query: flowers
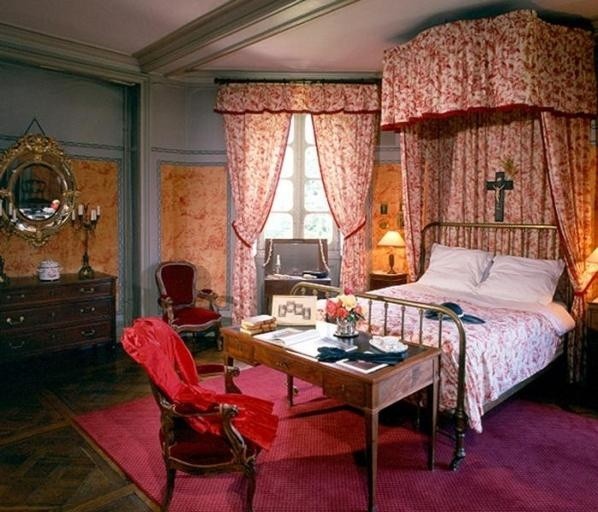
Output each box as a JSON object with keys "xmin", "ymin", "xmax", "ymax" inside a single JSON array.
[{"xmin": 324, "ymin": 287, "xmax": 365, "ymax": 321}]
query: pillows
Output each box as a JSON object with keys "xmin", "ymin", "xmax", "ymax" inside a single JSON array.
[
  {"xmin": 478, "ymin": 251, "xmax": 567, "ymax": 309},
  {"xmin": 414, "ymin": 241, "xmax": 492, "ymax": 292}
]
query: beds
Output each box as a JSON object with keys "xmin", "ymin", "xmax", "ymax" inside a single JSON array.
[{"xmin": 290, "ymin": 220, "xmax": 576, "ymax": 472}]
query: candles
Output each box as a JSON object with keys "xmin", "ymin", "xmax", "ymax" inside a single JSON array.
[
  {"xmin": 90, "ymin": 209, "xmax": 96, "ymax": 219},
  {"xmin": 96, "ymin": 205, "xmax": 100, "ymax": 215},
  {"xmin": 70, "ymin": 208, "xmax": 76, "ymax": 221},
  {"xmin": 78, "ymin": 205, "xmax": 83, "ymax": 215}
]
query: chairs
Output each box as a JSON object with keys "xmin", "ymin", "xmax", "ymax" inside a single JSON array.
[
  {"xmin": 122, "ymin": 316, "xmax": 276, "ymax": 511},
  {"xmin": 152, "ymin": 260, "xmax": 227, "ymax": 351}
]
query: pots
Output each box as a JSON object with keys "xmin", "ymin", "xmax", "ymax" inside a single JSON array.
[{"xmin": 35, "ymin": 259, "xmax": 63, "ymax": 282}]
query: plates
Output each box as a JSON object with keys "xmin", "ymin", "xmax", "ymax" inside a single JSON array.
[{"xmin": 368, "ymin": 335, "xmax": 408, "ymax": 353}]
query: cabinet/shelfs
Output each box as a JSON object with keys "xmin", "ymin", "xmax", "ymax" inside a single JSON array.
[
  {"xmin": 2, "ymin": 271, "xmax": 118, "ymax": 360},
  {"xmin": 263, "ymin": 240, "xmax": 331, "ymax": 314}
]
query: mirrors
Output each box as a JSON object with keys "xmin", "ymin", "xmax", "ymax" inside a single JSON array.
[{"xmin": 1, "ymin": 133, "xmax": 78, "ymax": 245}]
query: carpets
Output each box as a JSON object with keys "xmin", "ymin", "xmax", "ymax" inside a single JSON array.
[{"xmin": 76, "ymin": 359, "xmax": 598, "ymax": 510}]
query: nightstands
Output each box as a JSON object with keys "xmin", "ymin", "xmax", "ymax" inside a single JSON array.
[
  {"xmin": 369, "ymin": 272, "xmax": 406, "ymax": 291},
  {"xmin": 580, "ymin": 302, "xmax": 598, "ymax": 413}
]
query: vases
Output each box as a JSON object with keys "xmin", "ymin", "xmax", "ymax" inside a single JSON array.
[{"xmin": 333, "ymin": 316, "xmax": 356, "ymax": 335}]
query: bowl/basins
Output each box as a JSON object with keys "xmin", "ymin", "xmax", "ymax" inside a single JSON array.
[{"xmin": 383, "ymin": 335, "xmax": 399, "ymax": 348}]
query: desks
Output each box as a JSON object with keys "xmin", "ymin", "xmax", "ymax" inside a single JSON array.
[{"xmin": 224, "ymin": 316, "xmax": 443, "ymax": 510}]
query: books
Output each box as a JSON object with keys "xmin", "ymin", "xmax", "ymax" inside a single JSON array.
[
  {"xmin": 241, "ymin": 323, "xmax": 276, "ymax": 330},
  {"xmin": 241, "ymin": 314, "xmax": 276, "ymax": 326},
  {"xmin": 240, "ymin": 327, "xmax": 277, "ymax": 336}
]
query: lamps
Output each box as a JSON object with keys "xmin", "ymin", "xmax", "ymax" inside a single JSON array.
[
  {"xmin": 377, "ymin": 229, "xmax": 408, "ymax": 275},
  {"xmin": 588, "ymin": 238, "xmax": 598, "ymax": 296}
]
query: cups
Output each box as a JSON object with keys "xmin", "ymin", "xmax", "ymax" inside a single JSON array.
[{"xmin": 372, "ymin": 336, "xmax": 383, "ymax": 346}]
